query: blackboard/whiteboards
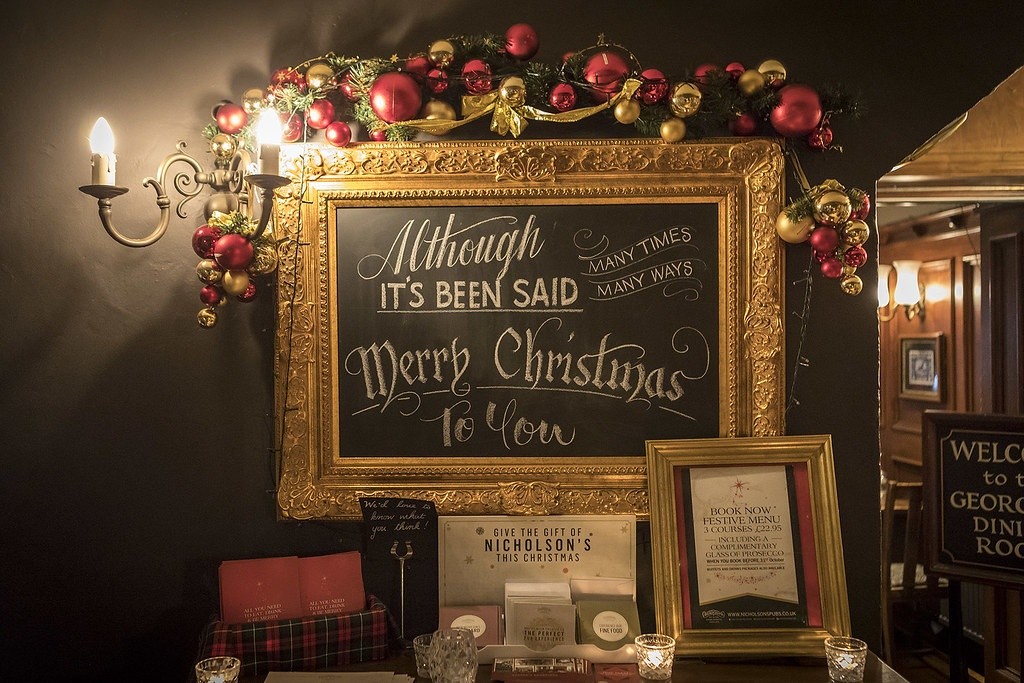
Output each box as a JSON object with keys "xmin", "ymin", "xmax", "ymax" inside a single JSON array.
[{"xmin": 276, "ymin": 139, "xmax": 790, "ymax": 523}]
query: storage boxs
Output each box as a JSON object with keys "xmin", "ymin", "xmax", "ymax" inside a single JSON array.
[{"xmin": 191, "ymin": 599, "xmax": 404, "ymax": 683}]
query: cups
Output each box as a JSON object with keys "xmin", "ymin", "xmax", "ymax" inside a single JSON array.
[
  {"xmin": 196, "ymin": 656, "xmax": 240, "ymax": 683},
  {"xmin": 635, "ymin": 633, "xmax": 675, "ymax": 680},
  {"xmin": 824, "ymin": 636, "xmax": 868, "ymax": 683},
  {"xmin": 413, "ymin": 634, "xmax": 436, "ymax": 679},
  {"xmin": 429, "ymin": 628, "xmax": 478, "ymax": 683}
]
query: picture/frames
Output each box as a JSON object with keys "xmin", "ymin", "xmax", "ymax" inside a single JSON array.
[
  {"xmin": 646, "ymin": 440, "xmax": 853, "ymax": 661},
  {"xmin": 922, "ymin": 409, "xmax": 1024, "ymax": 591},
  {"xmin": 259, "ymin": 132, "xmax": 790, "ymax": 519},
  {"xmin": 896, "ymin": 328, "xmax": 946, "ymax": 404}
]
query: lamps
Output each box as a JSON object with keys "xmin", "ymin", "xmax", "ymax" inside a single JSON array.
[
  {"xmin": 78, "ymin": 98, "xmax": 294, "ymax": 246},
  {"xmin": 874, "ymin": 256, "xmax": 928, "ymax": 321}
]
